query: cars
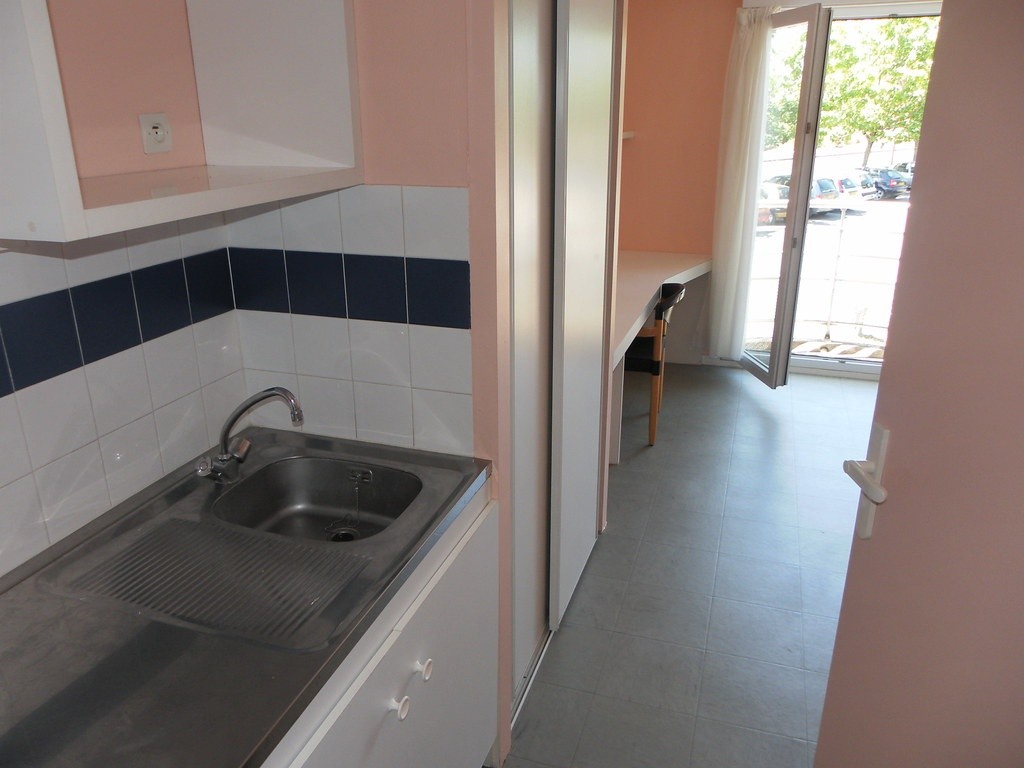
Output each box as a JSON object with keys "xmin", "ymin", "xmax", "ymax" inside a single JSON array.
[{"xmin": 754, "ymin": 159, "xmax": 916, "ymax": 227}]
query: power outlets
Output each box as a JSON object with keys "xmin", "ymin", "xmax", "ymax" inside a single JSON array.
[{"xmin": 139, "ymin": 108, "xmax": 173, "ymax": 156}]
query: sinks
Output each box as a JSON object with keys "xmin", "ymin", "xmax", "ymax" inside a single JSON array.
[{"xmin": 210, "ymin": 456, "xmax": 423, "ymax": 544}]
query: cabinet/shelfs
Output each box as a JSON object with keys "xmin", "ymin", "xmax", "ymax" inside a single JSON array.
[
  {"xmin": 257, "ymin": 482, "xmax": 501, "ymax": 767},
  {"xmin": 0, "ymin": 0, "xmax": 363, "ymax": 242}
]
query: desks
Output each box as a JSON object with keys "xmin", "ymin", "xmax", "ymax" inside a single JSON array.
[{"xmin": 610, "ymin": 249, "xmax": 714, "ymax": 464}]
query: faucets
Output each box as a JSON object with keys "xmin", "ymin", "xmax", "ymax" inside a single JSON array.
[{"xmin": 194, "ymin": 386, "xmax": 305, "ymax": 486}]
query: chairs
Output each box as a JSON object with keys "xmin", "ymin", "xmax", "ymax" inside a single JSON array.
[{"xmin": 624, "ymin": 282, "xmax": 686, "ymax": 446}]
